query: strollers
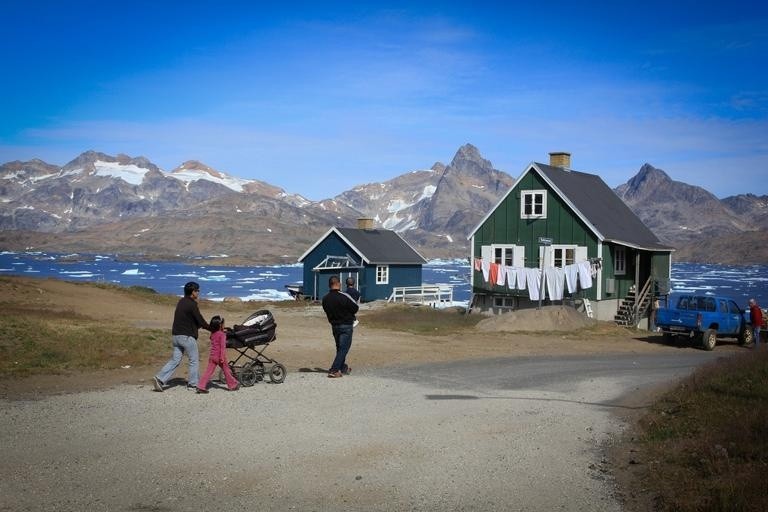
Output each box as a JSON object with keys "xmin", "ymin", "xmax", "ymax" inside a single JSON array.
[{"xmin": 219, "ymin": 310, "xmax": 286, "ymax": 387}]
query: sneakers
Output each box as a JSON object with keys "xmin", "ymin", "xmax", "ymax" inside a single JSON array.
[
  {"xmin": 328, "ymin": 371, "xmax": 342, "ymax": 377},
  {"xmin": 187, "ymin": 383, "xmax": 197, "ymax": 390},
  {"xmin": 346, "ymin": 368, "xmax": 351, "ymax": 373},
  {"xmin": 353, "ymin": 320, "xmax": 359, "ymax": 328},
  {"xmin": 153, "ymin": 376, "xmax": 162, "ymax": 391},
  {"xmin": 197, "ymin": 388, "xmax": 209, "ymax": 393},
  {"xmin": 234, "ymin": 385, "xmax": 239, "ymax": 391}
]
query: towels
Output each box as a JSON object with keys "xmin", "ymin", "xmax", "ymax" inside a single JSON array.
[{"xmin": 474, "ymin": 258, "xmax": 594, "ymax": 301}]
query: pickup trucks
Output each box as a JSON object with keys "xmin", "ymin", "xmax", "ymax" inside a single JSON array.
[{"xmin": 654, "ymin": 294, "xmax": 754, "ymax": 350}]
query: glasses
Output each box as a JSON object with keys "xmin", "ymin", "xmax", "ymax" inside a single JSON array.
[{"xmin": 195, "ymin": 289, "xmax": 200, "ymax": 292}]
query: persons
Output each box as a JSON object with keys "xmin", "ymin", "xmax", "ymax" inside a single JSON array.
[
  {"xmin": 322, "ymin": 275, "xmax": 359, "ymax": 378},
  {"xmin": 344, "ymin": 277, "xmax": 359, "ymax": 327},
  {"xmin": 196, "ymin": 315, "xmax": 241, "ymax": 392},
  {"xmin": 152, "ymin": 281, "xmax": 210, "ymax": 392},
  {"xmin": 749, "ymin": 298, "xmax": 763, "ymax": 346}
]
